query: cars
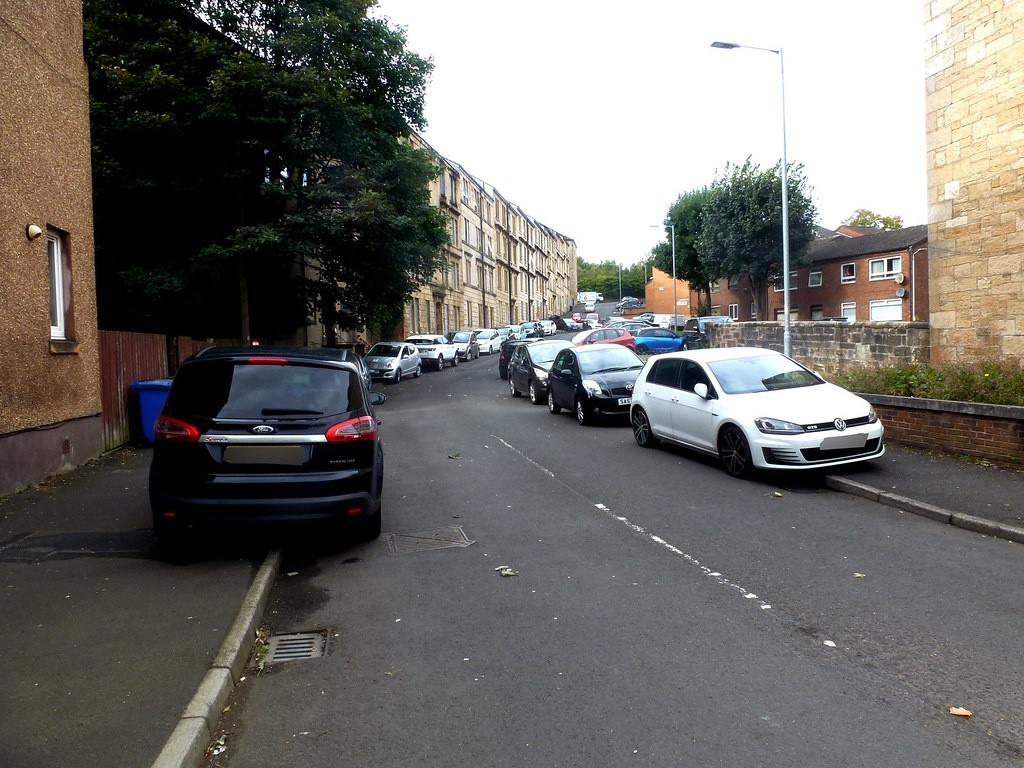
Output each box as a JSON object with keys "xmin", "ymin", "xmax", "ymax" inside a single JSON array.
[
  {"xmin": 519, "ymin": 321, "xmax": 544, "ymax": 338},
  {"xmin": 570, "ymin": 327, "xmax": 635, "ymax": 352},
  {"xmin": 630, "ymin": 346, "xmax": 887, "ymax": 477},
  {"xmin": 363, "ymin": 341, "xmax": 423, "ymax": 384},
  {"xmin": 581, "ymin": 318, "xmax": 604, "ymax": 330},
  {"xmin": 634, "ymin": 327, "xmax": 689, "ymax": 354},
  {"xmin": 507, "ymin": 338, "xmax": 576, "ymax": 404},
  {"xmin": 632, "ymin": 312, "xmax": 654, "ymax": 322},
  {"xmin": 556, "ymin": 319, "xmax": 581, "ymax": 332},
  {"xmin": 614, "ymin": 296, "xmax": 641, "ymax": 311},
  {"xmin": 499, "ymin": 337, "xmax": 547, "ymax": 380},
  {"xmin": 540, "ymin": 320, "xmax": 557, "ymax": 336},
  {"xmin": 601, "ymin": 315, "xmax": 660, "ymax": 331},
  {"xmin": 505, "ymin": 325, "xmax": 527, "ymax": 339},
  {"xmin": 572, "ymin": 312, "xmax": 581, "ymax": 319},
  {"xmin": 472, "ymin": 329, "xmax": 509, "ymax": 354},
  {"xmin": 445, "ymin": 332, "xmax": 481, "ymax": 362},
  {"xmin": 546, "ymin": 343, "xmax": 646, "ymax": 426}
]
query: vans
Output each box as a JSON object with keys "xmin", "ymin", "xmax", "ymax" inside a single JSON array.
[{"xmin": 683, "ymin": 316, "xmax": 734, "ymax": 338}]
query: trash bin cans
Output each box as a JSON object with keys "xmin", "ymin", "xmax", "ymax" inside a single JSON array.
[{"xmin": 131, "ymin": 378, "xmax": 173, "ymax": 446}]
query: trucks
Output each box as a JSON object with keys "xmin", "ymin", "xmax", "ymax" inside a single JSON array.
[
  {"xmin": 577, "ymin": 291, "xmax": 604, "ymax": 306},
  {"xmin": 649, "ymin": 313, "xmax": 684, "ymax": 331}
]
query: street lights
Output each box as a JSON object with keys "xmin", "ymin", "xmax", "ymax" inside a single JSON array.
[
  {"xmin": 911, "ymin": 248, "xmax": 928, "ymax": 322},
  {"xmin": 711, "ymin": 36, "xmax": 794, "ymax": 360},
  {"xmin": 649, "ymin": 224, "xmax": 677, "ymax": 336},
  {"xmin": 642, "ymin": 263, "xmax": 647, "ymax": 284}
]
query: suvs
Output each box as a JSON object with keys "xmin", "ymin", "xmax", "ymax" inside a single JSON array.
[
  {"xmin": 404, "ymin": 334, "xmax": 459, "ymax": 372},
  {"xmin": 147, "ymin": 344, "xmax": 385, "ymax": 545}
]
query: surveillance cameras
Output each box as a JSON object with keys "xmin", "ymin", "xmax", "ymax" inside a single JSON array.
[{"xmin": 28, "ymin": 225, "xmax": 42, "ymax": 239}]
